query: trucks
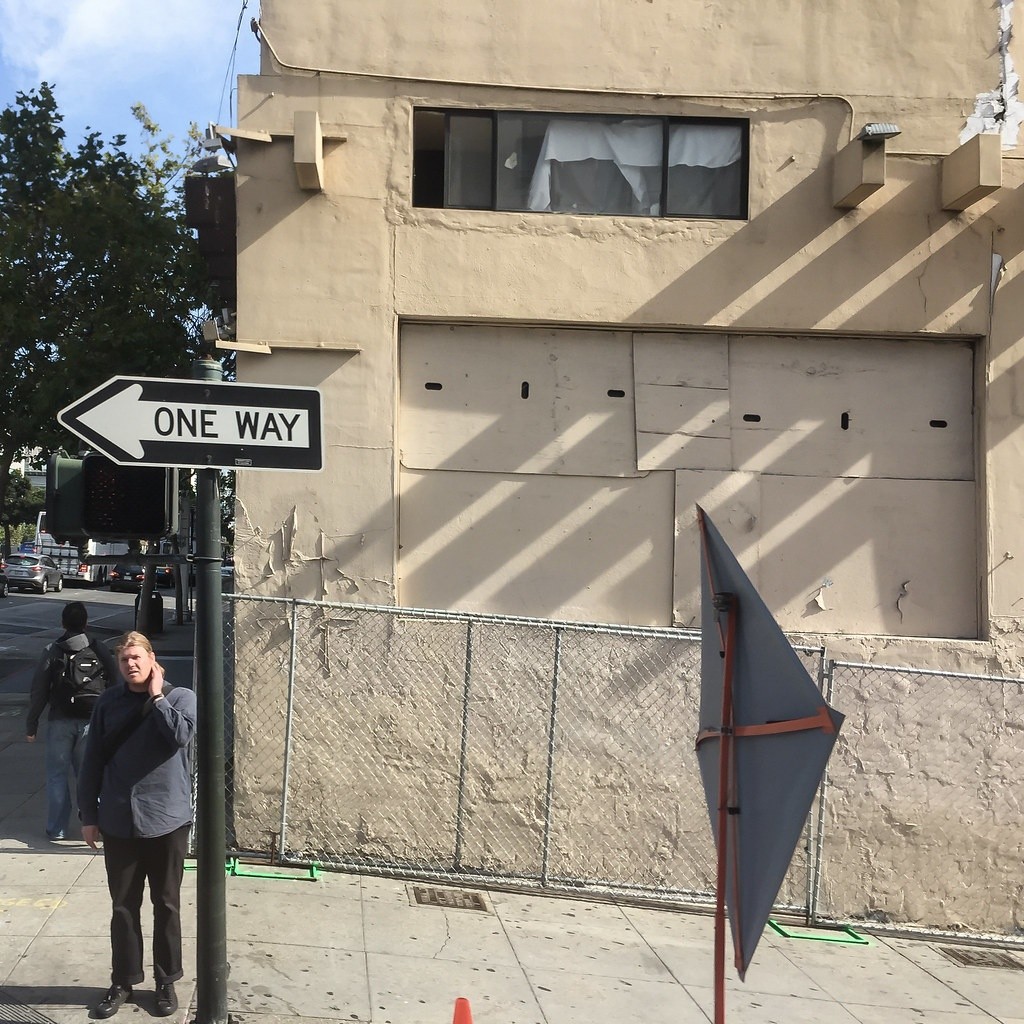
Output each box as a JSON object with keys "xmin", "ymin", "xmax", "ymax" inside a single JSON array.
[{"xmin": 34, "ymin": 511, "xmax": 147, "ymax": 588}]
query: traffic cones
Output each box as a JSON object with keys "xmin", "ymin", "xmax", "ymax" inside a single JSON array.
[{"xmin": 452, "ymin": 998, "xmax": 474, "ymax": 1024}]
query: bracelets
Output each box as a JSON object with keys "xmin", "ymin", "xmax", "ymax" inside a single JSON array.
[{"xmin": 150, "ymin": 693, "xmax": 164, "ymax": 703}]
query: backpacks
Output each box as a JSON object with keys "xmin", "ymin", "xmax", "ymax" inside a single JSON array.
[{"xmin": 53, "ymin": 638, "xmax": 107, "ymax": 712}]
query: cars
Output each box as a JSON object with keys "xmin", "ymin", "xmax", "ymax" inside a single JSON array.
[
  {"xmin": 155, "ymin": 563, "xmax": 177, "ymax": 590},
  {"xmin": 2, "ymin": 553, "xmax": 64, "ymax": 595},
  {"xmin": 110, "ymin": 562, "xmax": 159, "ymax": 593},
  {"xmin": 17, "ymin": 542, "xmax": 34, "ymax": 553},
  {"xmin": 222, "ymin": 557, "xmax": 235, "ymax": 578},
  {"xmin": 0, "ymin": 568, "xmax": 10, "ymax": 598}
]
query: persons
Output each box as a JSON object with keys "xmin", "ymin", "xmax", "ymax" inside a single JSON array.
[
  {"xmin": 79, "ymin": 632, "xmax": 198, "ymax": 1017},
  {"xmin": 26, "ymin": 602, "xmax": 115, "ymax": 840}
]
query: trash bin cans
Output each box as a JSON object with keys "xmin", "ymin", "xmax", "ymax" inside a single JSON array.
[{"xmin": 131, "ymin": 590, "xmax": 163, "ymax": 639}]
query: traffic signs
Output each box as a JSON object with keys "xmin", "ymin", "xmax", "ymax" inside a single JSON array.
[{"xmin": 58, "ymin": 375, "xmax": 326, "ymax": 476}]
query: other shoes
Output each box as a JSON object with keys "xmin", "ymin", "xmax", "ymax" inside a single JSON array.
[
  {"xmin": 155, "ymin": 981, "xmax": 178, "ymax": 1015},
  {"xmin": 96, "ymin": 984, "xmax": 133, "ymax": 1017},
  {"xmin": 46, "ymin": 828, "xmax": 64, "ymax": 840}
]
